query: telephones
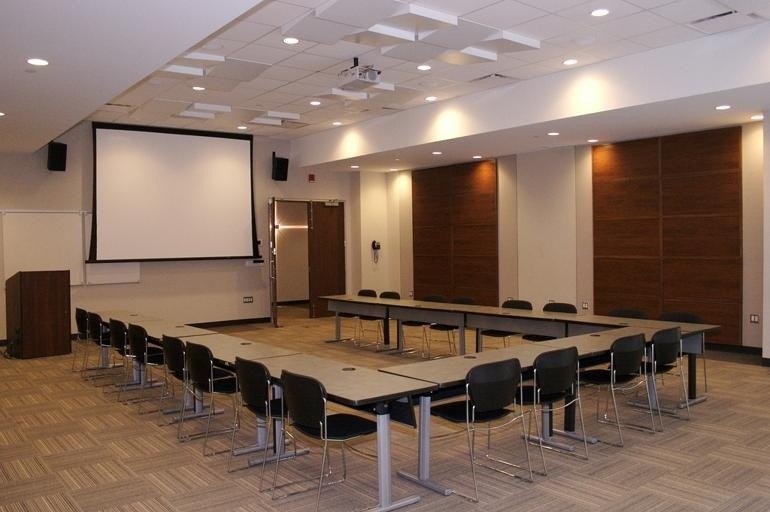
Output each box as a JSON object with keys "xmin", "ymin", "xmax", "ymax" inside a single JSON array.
[{"xmin": 372, "ymin": 240, "xmax": 380, "ymax": 249}]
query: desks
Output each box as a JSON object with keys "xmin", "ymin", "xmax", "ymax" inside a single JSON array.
[
  {"xmin": 378, "ymin": 341, "xmax": 573, "ymax": 497},
  {"xmin": 248, "ymin": 352, "xmax": 440, "ymax": 510}
]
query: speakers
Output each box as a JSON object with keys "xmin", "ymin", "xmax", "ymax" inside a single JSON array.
[
  {"xmin": 272, "ymin": 158, "xmax": 288, "ymax": 181},
  {"xmin": 47, "ymin": 142, "xmax": 67, "ymax": 171}
]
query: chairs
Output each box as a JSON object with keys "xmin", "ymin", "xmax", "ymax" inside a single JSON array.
[
  {"xmin": 230, "ymin": 356, "xmax": 331, "ymax": 493},
  {"xmin": 533, "ymin": 316, "xmax": 720, "ymax": 448},
  {"xmin": 606, "ymin": 307, "xmax": 646, "ymax": 319},
  {"xmin": 488, "ymin": 347, "xmax": 591, "ymax": 478},
  {"xmin": 319, "ymin": 290, "xmax": 577, "ymax": 359},
  {"xmin": 656, "ymin": 310, "xmax": 712, "ymax": 402},
  {"xmin": 430, "ymin": 357, "xmax": 534, "ymax": 503},
  {"xmin": 270, "ymin": 368, "xmax": 379, "ymax": 508},
  {"xmin": 72, "ymin": 309, "xmax": 302, "ymax": 473}
]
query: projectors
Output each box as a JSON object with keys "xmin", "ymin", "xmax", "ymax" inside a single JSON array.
[{"xmin": 337, "ymin": 66, "xmax": 380, "ymax": 91}]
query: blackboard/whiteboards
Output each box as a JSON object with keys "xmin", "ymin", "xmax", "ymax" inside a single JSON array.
[{"xmin": 2, "ymin": 209, "xmax": 144, "ymax": 289}]
